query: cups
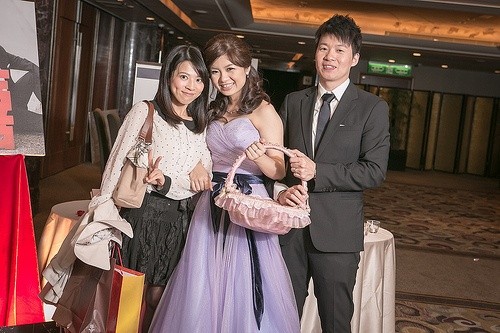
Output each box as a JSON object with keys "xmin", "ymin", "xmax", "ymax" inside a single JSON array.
[
  {"xmin": 367, "ymin": 220, "xmax": 380, "ymax": 233},
  {"xmin": 364, "ymin": 223, "xmax": 369, "ymax": 236}
]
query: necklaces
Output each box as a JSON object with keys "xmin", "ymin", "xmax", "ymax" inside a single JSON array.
[{"xmin": 225, "ymin": 109, "xmax": 239, "ymax": 114}]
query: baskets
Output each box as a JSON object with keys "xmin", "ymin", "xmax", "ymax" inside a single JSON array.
[{"xmin": 214, "ymin": 143, "xmax": 312, "ymax": 236}]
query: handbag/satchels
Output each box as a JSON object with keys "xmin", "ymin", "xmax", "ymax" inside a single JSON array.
[
  {"xmin": 112, "ymin": 99, "xmax": 155, "ymax": 209},
  {"xmin": 106, "ymin": 242, "xmax": 145, "ymax": 333},
  {"xmin": 52, "ymin": 243, "xmax": 116, "ymax": 333}
]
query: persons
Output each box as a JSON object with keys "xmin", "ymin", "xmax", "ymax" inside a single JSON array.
[
  {"xmin": 1, "ymin": 46, "xmax": 43, "ymax": 134},
  {"xmin": 148, "ymin": 34, "xmax": 301, "ymax": 333},
  {"xmin": 88, "ymin": 45, "xmax": 213, "ymax": 333},
  {"xmin": 262, "ymin": 13, "xmax": 390, "ymax": 333}
]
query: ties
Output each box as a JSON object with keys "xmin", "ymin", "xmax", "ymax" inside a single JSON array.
[{"xmin": 314, "ymin": 93, "xmax": 335, "ymax": 159}]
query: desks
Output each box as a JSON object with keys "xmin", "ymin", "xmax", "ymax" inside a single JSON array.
[
  {"xmin": 300, "ymin": 224, "xmax": 395, "ymax": 333},
  {"xmin": 37, "ymin": 200, "xmax": 92, "ymax": 291}
]
